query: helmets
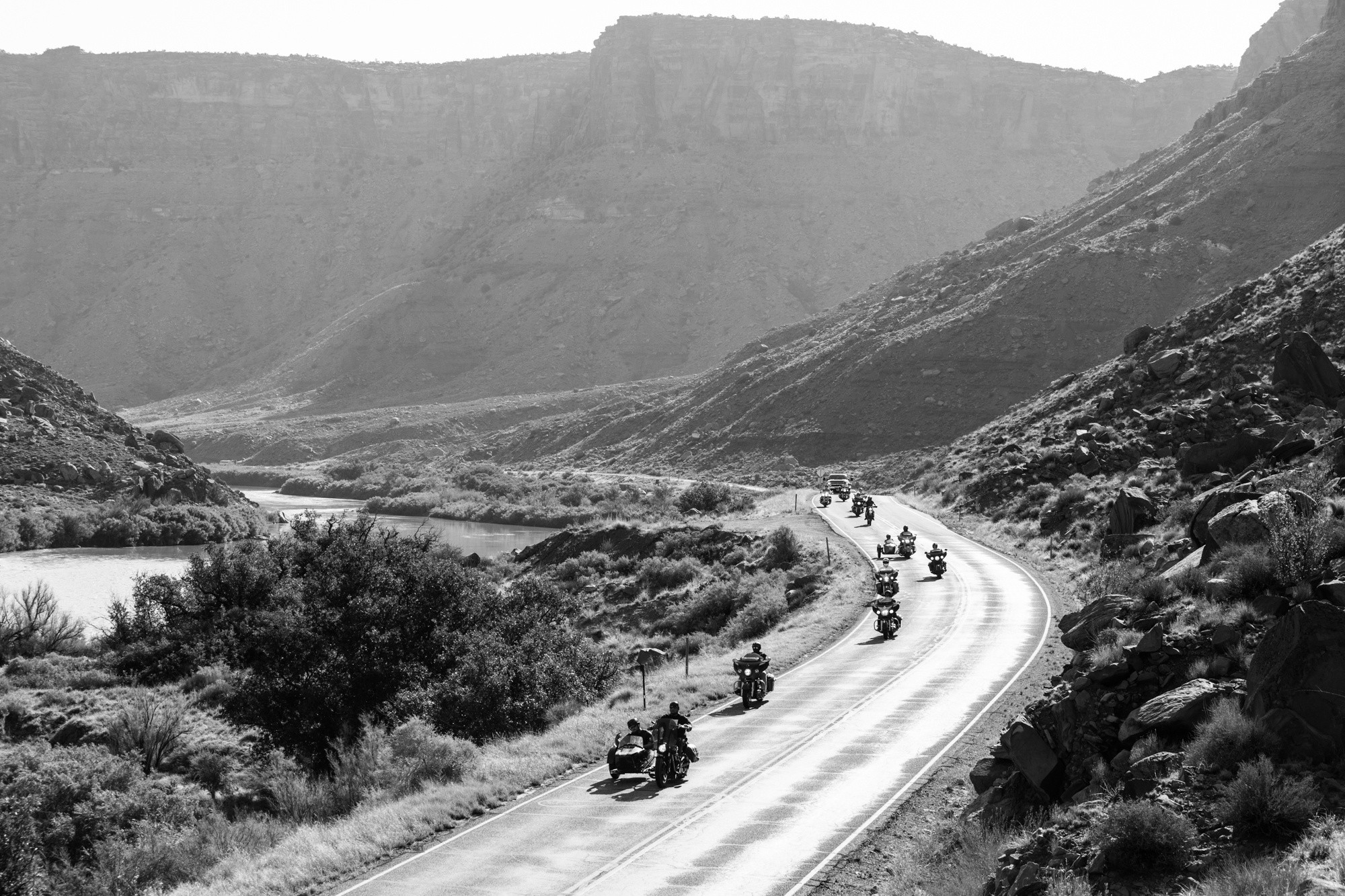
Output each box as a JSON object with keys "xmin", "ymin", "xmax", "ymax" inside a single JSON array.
[
  {"xmin": 628, "ymin": 718, "xmax": 638, "ymax": 728},
  {"xmin": 933, "ymin": 543, "xmax": 938, "ymax": 548},
  {"xmin": 885, "ymin": 590, "xmax": 892, "ymax": 595},
  {"xmin": 670, "ymin": 701, "xmax": 679, "ymax": 706},
  {"xmin": 903, "ymin": 525, "xmax": 908, "ymax": 530},
  {"xmin": 752, "ymin": 642, "xmax": 761, "ymax": 647},
  {"xmin": 883, "ymin": 558, "xmax": 889, "ymax": 561},
  {"xmin": 868, "ymin": 495, "xmax": 872, "ymax": 500},
  {"xmin": 857, "ymin": 492, "xmax": 860, "ymax": 496},
  {"xmin": 886, "ymin": 534, "xmax": 890, "ymax": 537}
]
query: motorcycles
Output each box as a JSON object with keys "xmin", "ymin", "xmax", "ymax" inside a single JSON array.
[
  {"xmin": 877, "ymin": 534, "xmax": 917, "ymax": 559},
  {"xmin": 924, "ymin": 548, "xmax": 947, "ymax": 579},
  {"xmin": 607, "ymin": 721, "xmax": 699, "ymax": 787},
  {"xmin": 864, "ymin": 505, "xmax": 877, "ymax": 526},
  {"xmin": 872, "ymin": 596, "xmax": 901, "ymax": 640},
  {"xmin": 851, "ymin": 492, "xmax": 868, "ymax": 517},
  {"xmin": 820, "ymin": 490, "xmax": 832, "ymax": 508},
  {"xmin": 732, "ymin": 652, "xmax": 777, "ymax": 709},
  {"xmin": 839, "ymin": 486, "xmax": 850, "ymax": 502},
  {"xmin": 875, "ymin": 569, "xmax": 900, "ymax": 598}
]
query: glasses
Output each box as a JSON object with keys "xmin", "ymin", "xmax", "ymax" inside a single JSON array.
[
  {"xmin": 669, "ymin": 706, "xmax": 677, "ymax": 708},
  {"xmin": 753, "ymin": 646, "xmax": 759, "ymax": 649},
  {"xmin": 629, "ymin": 725, "xmax": 637, "ymax": 729}
]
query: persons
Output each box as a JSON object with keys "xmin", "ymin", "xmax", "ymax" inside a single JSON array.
[
  {"xmin": 856, "ymin": 488, "xmax": 865, "ymax": 497},
  {"xmin": 646, "ymin": 700, "xmax": 692, "ymax": 765},
  {"xmin": 872, "ymin": 590, "xmax": 901, "ymax": 632},
  {"xmin": 734, "ymin": 642, "xmax": 770, "ymax": 697},
  {"xmin": 852, "ymin": 493, "xmax": 863, "ymax": 505},
  {"xmin": 614, "ymin": 717, "xmax": 654, "ymax": 759},
  {"xmin": 820, "ymin": 484, "xmax": 831, "ymax": 504},
  {"xmin": 875, "ymin": 557, "xmax": 900, "ymax": 592},
  {"xmin": 928, "ymin": 543, "xmax": 947, "ymax": 572},
  {"xmin": 898, "ymin": 525, "xmax": 916, "ymax": 554},
  {"xmin": 864, "ymin": 495, "xmax": 876, "ymax": 521},
  {"xmin": 885, "ymin": 534, "xmax": 894, "ymax": 544},
  {"xmin": 839, "ymin": 481, "xmax": 852, "ymax": 493}
]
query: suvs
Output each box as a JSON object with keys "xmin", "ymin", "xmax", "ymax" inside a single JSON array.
[{"xmin": 823, "ymin": 473, "xmax": 853, "ymax": 494}]
